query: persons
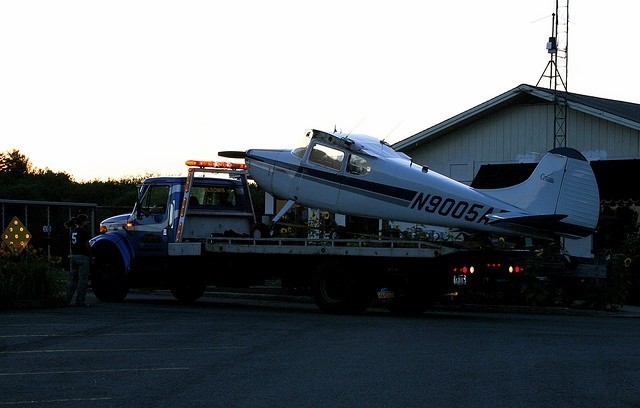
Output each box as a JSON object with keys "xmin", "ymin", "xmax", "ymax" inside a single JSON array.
[
  {"xmin": 64, "ymin": 213, "xmax": 91, "ymax": 308},
  {"xmin": 218, "ymin": 190, "xmax": 233, "ymax": 206}
]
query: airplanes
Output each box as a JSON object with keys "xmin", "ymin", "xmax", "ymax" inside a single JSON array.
[{"xmin": 245, "ymin": 127, "xmax": 601, "ymax": 248}]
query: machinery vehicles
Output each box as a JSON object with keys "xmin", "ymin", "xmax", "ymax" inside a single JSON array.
[{"xmin": 88, "ymin": 160, "xmax": 528, "ymax": 314}]
